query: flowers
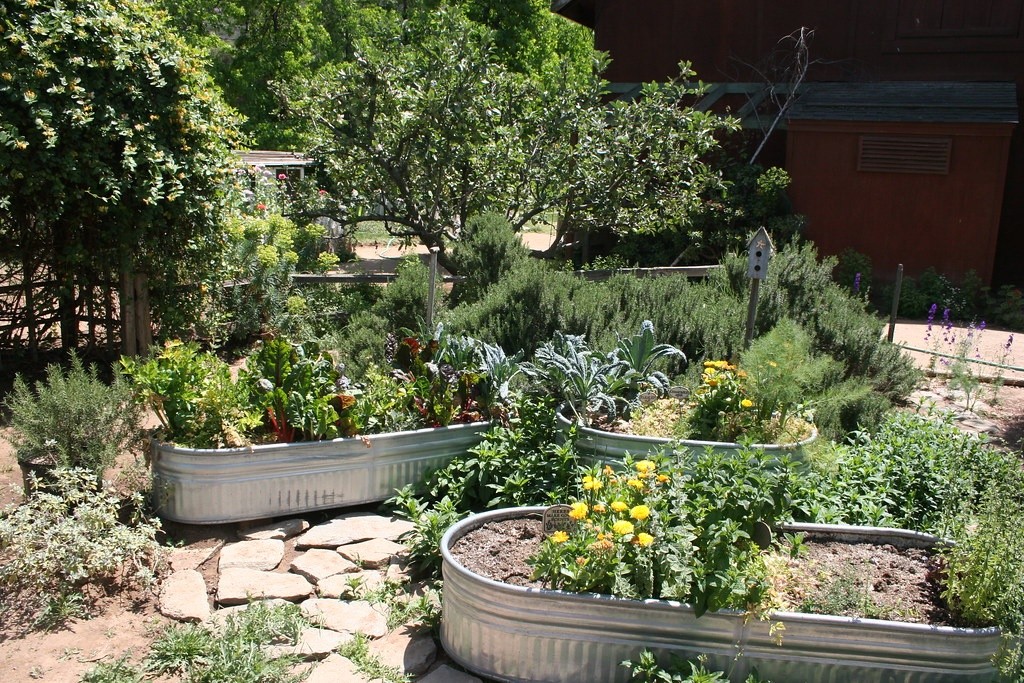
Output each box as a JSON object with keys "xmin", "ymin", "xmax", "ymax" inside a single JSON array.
[{"xmin": 133, "ymin": 312, "xmax": 803, "ymax": 635}]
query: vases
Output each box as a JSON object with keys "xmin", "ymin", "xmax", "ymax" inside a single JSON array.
[
  {"xmin": 439, "ymin": 504, "xmax": 1024, "ymax": 683},
  {"xmin": 556, "ymin": 390, "xmax": 819, "ymax": 476},
  {"xmin": 143, "ymin": 416, "xmax": 494, "ymax": 528}
]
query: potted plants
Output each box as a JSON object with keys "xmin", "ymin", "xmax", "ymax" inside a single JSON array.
[{"xmin": 7, "ymin": 353, "xmax": 122, "ymax": 518}]
